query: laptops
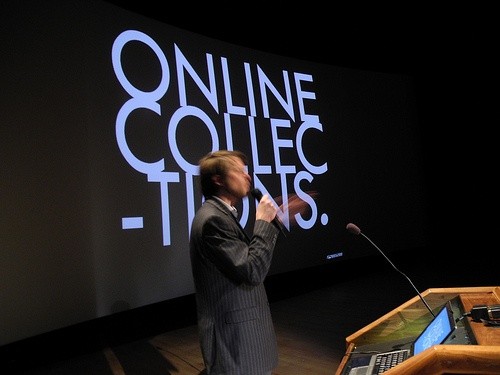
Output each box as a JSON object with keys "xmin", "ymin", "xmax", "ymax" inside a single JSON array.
[{"xmin": 366, "ymin": 302, "xmax": 457, "ymax": 375}]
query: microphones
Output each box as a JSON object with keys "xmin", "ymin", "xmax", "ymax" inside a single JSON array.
[
  {"xmin": 345, "ymin": 222, "xmax": 436, "ymax": 318},
  {"xmin": 251, "ymin": 189, "xmax": 288, "ymax": 239}
]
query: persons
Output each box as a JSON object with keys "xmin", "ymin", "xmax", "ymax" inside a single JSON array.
[{"xmin": 188, "ymin": 149, "xmax": 320, "ymax": 375}]
